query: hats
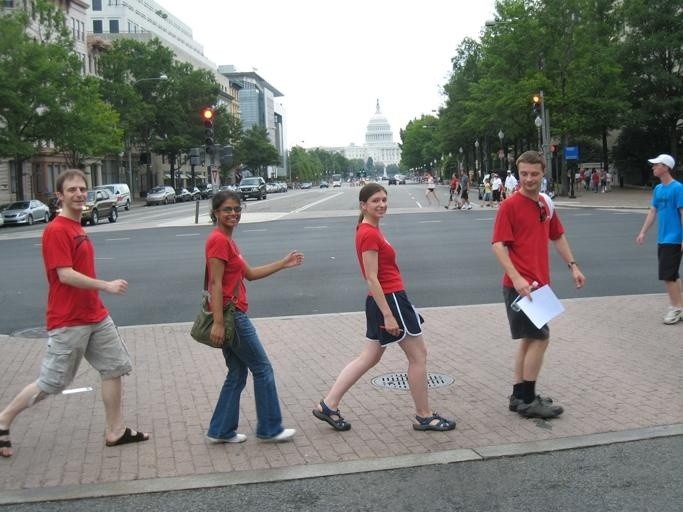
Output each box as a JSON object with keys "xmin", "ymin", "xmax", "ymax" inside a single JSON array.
[{"xmin": 647, "ymin": 154, "xmax": 676, "ymax": 170}]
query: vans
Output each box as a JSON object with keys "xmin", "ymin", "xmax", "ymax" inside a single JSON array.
[{"xmin": 94, "ymin": 184, "xmax": 130, "ymax": 212}]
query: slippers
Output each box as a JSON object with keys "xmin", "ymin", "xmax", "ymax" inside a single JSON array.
[
  {"xmin": 106, "ymin": 427, "xmax": 149, "ymax": 446},
  {"xmin": 0, "ymin": 429, "xmax": 12, "ymax": 457}
]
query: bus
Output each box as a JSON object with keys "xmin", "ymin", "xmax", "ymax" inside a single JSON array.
[
  {"xmin": 357, "ymin": 172, "xmax": 365, "ymax": 180},
  {"xmin": 331, "ymin": 174, "xmax": 341, "ymax": 187}
]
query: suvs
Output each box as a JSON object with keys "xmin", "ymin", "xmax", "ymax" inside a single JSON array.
[
  {"xmin": 236, "ymin": 177, "xmax": 266, "ymax": 201},
  {"xmin": 388, "ymin": 177, "xmax": 396, "ymax": 185},
  {"xmin": 58, "ymin": 188, "xmax": 118, "ymax": 225},
  {"xmin": 319, "ymin": 181, "xmax": 328, "ymax": 188},
  {"xmin": 197, "ymin": 183, "xmax": 212, "ymax": 199}
]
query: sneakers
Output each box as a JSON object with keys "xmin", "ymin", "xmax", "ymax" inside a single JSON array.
[
  {"xmin": 508, "ymin": 394, "xmax": 563, "ymax": 418},
  {"xmin": 664, "ymin": 308, "xmax": 683, "ymax": 324},
  {"xmin": 260, "ymin": 428, "xmax": 296, "ymax": 440},
  {"xmin": 207, "ymin": 434, "xmax": 247, "ymax": 443}
]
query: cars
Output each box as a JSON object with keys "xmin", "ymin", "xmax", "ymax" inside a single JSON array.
[
  {"xmin": 45, "ymin": 198, "xmax": 60, "ymax": 220},
  {"xmin": 266, "ymin": 182, "xmax": 287, "ymax": 193},
  {"xmin": 381, "ymin": 177, "xmax": 386, "ymax": 180},
  {"xmin": 366, "ymin": 178, "xmax": 369, "ymax": 181},
  {"xmin": 186, "ymin": 187, "xmax": 200, "ymax": 201},
  {"xmin": 300, "ymin": 183, "xmax": 311, "ymax": 189},
  {"xmin": 398, "ymin": 178, "xmax": 405, "ymax": 185},
  {"xmin": 174, "ymin": 189, "xmax": 192, "ymax": 202},
  {"xmin": 218, "ymin": 185, "xmax": 229, "ymax": 192},
  {"xmin": 229, "ymin": 183, "xmax": 238, "ymax": 191},
  {"xmin": 145, "ymin": 186, "xmax": 176, "ymax": 204},
  {"xmin": 0, "ymin": 200, "xmax": 49, "ymax": 226}
]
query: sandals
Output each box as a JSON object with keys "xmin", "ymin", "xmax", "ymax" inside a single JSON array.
[
  {"xmin": 312, "ymin": 399, "xmax": 351, "ymax": 431},
  {"xmin": 413, "ymin": 414, "xmax": 456, "ymax": 431}
]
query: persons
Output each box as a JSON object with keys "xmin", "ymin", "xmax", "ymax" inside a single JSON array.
[
  {"xmin": 312, "ymin": 182, "xmax": 455, "ymax": 432},
  {"xmin": 296, "ymin": 176, "xmax": 302, "ymax": 189},
  {"xmin": 414, "ymin": 169, "xmax": 556, "ymax": 210},
  {"xmin": 350, "ymin": 176, "xmax": 367, "ymax": 187},
  {"xmin": 634, "ymin": 153, "xmax": 683, "ymax": 325},
  {"xmin": 567, "ymin": 166, "xmax": 616, "ymax": 193},
  {"xmin": 0, "ymin": 169, "xmax": 150, "ymax": 458},
  {"xmin": 491, "ymin": 150, "xmax": 586, "ymax": 419},
  {"xmin": 203, "ymin": 189, "xmax": 305, "ymax": 444}
]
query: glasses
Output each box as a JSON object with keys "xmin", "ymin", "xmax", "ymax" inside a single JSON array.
[
  {"xmin": 215, "ymin": 206, "xmax": 242, "ymax": 212},
  {"xmin": 537, "ymin": 199, "xmax": 547, "ymax": 223}
]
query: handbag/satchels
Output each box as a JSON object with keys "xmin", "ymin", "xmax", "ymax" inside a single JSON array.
[{"xmin": 189, "ymin": 295, "xmax": 235, "ymax": 348}]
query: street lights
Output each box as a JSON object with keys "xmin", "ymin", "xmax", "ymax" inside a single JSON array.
[
  {"xmin": 421, "ymin": 125, "xmax": 436, "ymax": 130},
  {"xmin": 429, "ymin": 162, "xmax": 433, "ymax": 176},
  {"xmin": 229, "ymin": 109, "xmax": 243, "ymax": 116},
  {"xmin": 408, "ymin": 163, "xmax": 426, "ymax": 176},
  {"xmin": 440, "ymin": 156, "xmax": 443, "ymax": 176},
  {"xmin": 288, "ymin": 140, "xmax": 304, "ymax": 183},
  {"xmin": 125, "ymin": 75, "xmax": 169, "ymax": 192},
  {"xmin": 474, "ymin": 140, "xmax": 479, "ymax": 183},
  {"xmin": 497, "ymin": 130, "xmax": 504, "ymax": 170},
  {"xmin": 458, "ymin": 147, "xmax": 462, "ymax": 175},
  {"xmin": 448, "ymin": 152, "xmax": 451, "ymax": 179},
  {"xmin": 534, "ymin": 117, "xmax": 541, "ymax": 149},
  {"xmin": 433, "ymin": 159, "xmax": 437, "ymax": 178}
]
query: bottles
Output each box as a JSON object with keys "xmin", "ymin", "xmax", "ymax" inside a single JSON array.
[{"xmin": 508, "ymin": 280, "xmax": 541, "ymax": 312}]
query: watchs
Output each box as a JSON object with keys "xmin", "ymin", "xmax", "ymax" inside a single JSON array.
[{"xmin": 567, "ymin": 261, "xmax": 576, "ymax": 268}]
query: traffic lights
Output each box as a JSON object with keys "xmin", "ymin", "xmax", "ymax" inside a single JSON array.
[
  {"xmin": 203, "ymin": 109, "xmax": 213, "ymax": 146},
  {"xmin": 550, "ymin": 145, "xmax": 558, "ymax": 159},
  {"xmin": 533, "ymin": 97, "xmax": 540, "ymax": 114}
]
